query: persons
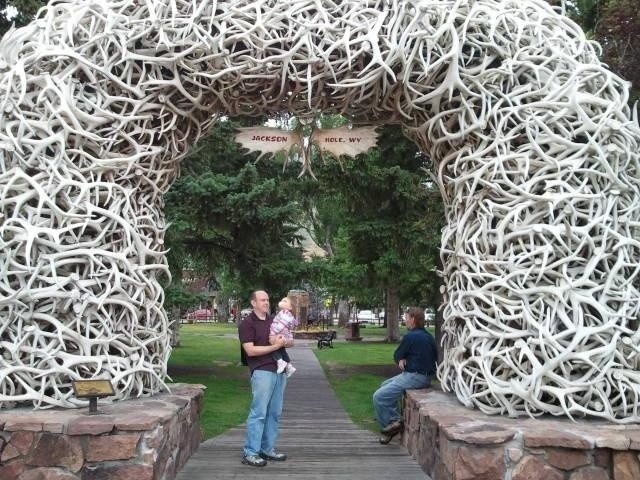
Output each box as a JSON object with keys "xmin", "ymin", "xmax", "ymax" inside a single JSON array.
[
  {"xmin": 269, "ymin": 294, "xmax": 300, "ymax": 379},
  {"xmin": 232, "ymin": 304, "xmax": 237, "ymax": 323},
  {"xmin": 371, "ymin": 306, "xmax": 439, "ymax": 446},
  {"xmin": 237, "ymin": 287, "xmax": 296, "ymax": 468}
]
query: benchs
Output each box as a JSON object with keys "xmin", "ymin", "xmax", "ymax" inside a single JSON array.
[{"xmin": 315, "ymin": 329, "xmax": 337, "ymax": 349}]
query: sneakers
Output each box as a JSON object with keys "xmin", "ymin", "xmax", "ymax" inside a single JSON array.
[
  {"xmin": 241, "ymin": 455, "xmax": 267, "ymax": 466},
  {"xmin": 260, "ymin": 448, "xmax": 287, "ymax": 461},
  {"xmin": 286, "ymin": 365, "xmax": 296, "ymax": 377},
  {"xmin": 277, "ymin": 360, "xmax": 287, "ymax": 374},
  {"xmin": 380, "ymin": 421, "xmax": 402, "ymax": 446}
]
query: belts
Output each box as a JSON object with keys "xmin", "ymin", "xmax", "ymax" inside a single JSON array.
[{"xmin": 408, "ymin": 369, "xmax": 431, "ymax": 376}]
